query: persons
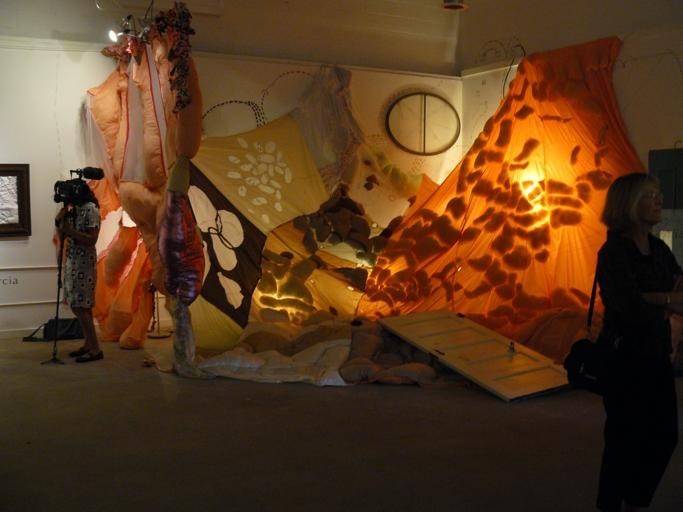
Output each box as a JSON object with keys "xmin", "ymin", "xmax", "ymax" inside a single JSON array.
[
  {"xmin": 60, "ymin": 179, "xmax": 105, "ymax": 365},
  {"xmin": 594, "ymin": 168, "xmax": 682, "ymax": 506},
  {"xmin": 47, "ymin": 180, "xmax": 68, "ymax": 307}
]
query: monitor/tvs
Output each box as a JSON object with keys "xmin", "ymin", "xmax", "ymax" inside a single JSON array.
[{"xmin": 648, "ymin": 148, "xmax": 683, "ymax": 208}]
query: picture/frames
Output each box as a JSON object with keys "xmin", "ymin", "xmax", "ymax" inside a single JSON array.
[{"xmin": 0, "ymin": 163, "xmax": 32, "ymax": 236}]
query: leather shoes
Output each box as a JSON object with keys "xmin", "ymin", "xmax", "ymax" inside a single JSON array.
[{"xmin": 66, "ymin": 345, "xmax": 104, "ymax": 362}]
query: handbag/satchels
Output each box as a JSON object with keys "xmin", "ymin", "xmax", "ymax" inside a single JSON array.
[{"xmin": 562, "ymin": 336, "xmax": 620, "ymax": 398}]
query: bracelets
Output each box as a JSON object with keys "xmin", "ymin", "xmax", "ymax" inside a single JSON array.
[{"xmin": 665, "ymin": 292, "xmax": 673, "ymax": 307}]
